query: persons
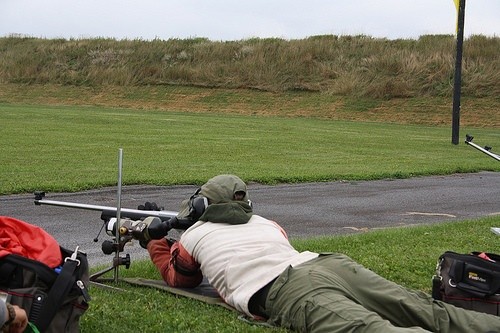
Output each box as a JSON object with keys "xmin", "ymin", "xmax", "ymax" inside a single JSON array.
[
  {"xmin": 133, "ymin": 174, "xmax": 500, "ymax": 333},
  {"xmin": 0, "ymin": 298, "xmax": 28, "ymax": 333}
]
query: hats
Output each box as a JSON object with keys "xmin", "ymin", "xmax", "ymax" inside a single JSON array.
[{"xmin": 195, "ymin": 173, "xmax": 248, "ymax": 204}]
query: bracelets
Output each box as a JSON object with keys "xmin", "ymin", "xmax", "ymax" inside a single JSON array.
[{"xmin": 2, "ymin": 303, "xmax": 16, "ymax": 328}]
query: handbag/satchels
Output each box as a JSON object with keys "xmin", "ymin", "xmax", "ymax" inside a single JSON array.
[
  {"xmin": 0, "ymin": 246, "xmax": 88, "ymax": 333},
  {"xmin": 432, "ymin": 250, "xmax": 500, "ymax": 319}
]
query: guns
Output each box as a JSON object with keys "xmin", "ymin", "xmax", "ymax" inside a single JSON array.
[
  {"xmin": 34, "ymin": 190, "xmax": 252, "ymax": 253},
  {"xmin": 464, "ymin": 132, "xmax": 500, "ymax": 161}
]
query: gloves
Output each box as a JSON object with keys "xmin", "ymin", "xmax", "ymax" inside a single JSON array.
[{"xmin": 137, "ymin": 202, "xmax": 167, "ymax": 223}]
